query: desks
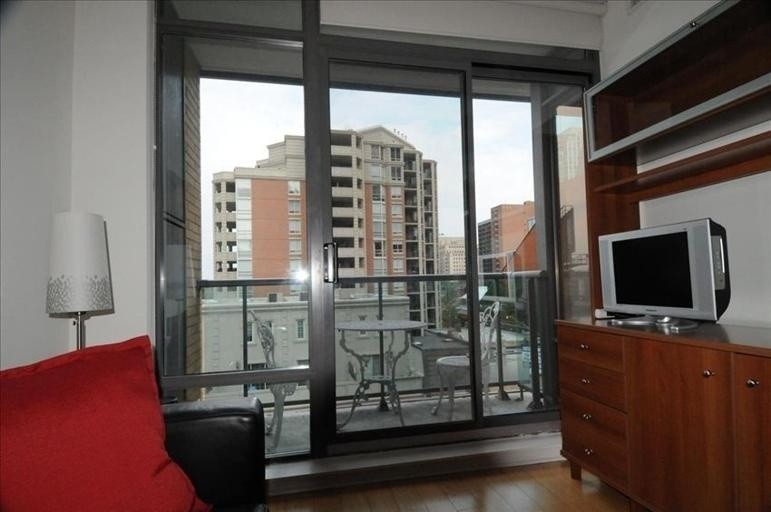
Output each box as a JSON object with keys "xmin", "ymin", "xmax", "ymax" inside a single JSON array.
[{"xmin": 336, "ymin": 318, "xmax": 426, "ymax": 429}]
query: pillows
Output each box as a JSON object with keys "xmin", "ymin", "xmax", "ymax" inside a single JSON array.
[{"xmin": 1, "ymin": 335, "xmax": 214, "ymax": 511}]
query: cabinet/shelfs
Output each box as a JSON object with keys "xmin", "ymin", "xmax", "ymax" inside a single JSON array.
[
  {"xmin": 555, "ymin": 312, "xmax": 771, "ymax": 511},
  {"xmin": 582, "ymin": 0, "xmax": 771, "ymax": 320}
]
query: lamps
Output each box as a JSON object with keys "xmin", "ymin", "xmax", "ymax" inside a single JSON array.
[{"xmin": 46, "ymin": 211, "xmax": 115, "ymax": 350}]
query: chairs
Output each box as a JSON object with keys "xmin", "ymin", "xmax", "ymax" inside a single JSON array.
[
  {"xmin": 432, "ymin": 301, "xmax": 500, "ymax": 422},
  {"xmin": 249, "ymin": 309, "xmax": 311, "ymax": 452}
]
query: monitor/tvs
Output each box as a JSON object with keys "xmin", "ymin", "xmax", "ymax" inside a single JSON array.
[{"xmin": 598, "ymin": 217, "xmax": 731, "ymax": 331}]
